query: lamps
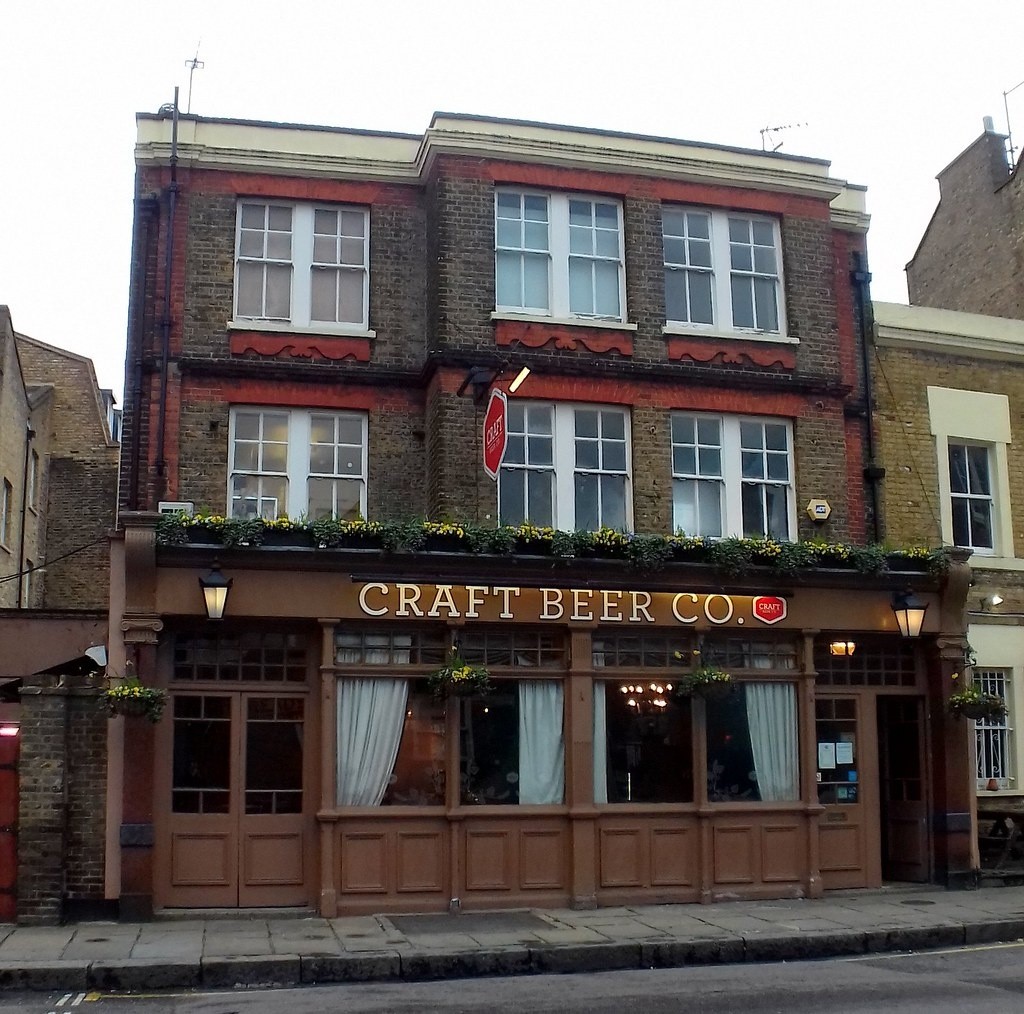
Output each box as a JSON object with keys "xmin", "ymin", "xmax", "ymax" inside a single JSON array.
[
  {"xmin": 197, "ymin": 555, "xmax": 234, "ymax": 628},
  {"xmin": 619, "ymin": 680, "xmax": 675, "ymax": 714},
  {"xmin": 829, "ymin": 639, "xmax": 855, "ymax": 657},
  {"xmin": 979, "ymin": 592, "xmax": 1005, "ymax": 611},
  {"xmin": 891, "ymin": 580, "xmax": 930, "ymax": 641}
]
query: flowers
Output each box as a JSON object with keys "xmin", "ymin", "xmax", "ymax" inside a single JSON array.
[
  {"xmin": 108, "ymin": 686, "xmax": 142, "ymax": 698},
  {"xmin": 443, "ymin": 666, "xmax": 475, "ymax": 683},
  {"xmin": 674, "ymin": 666, "xmax": 734, "ymax": 697},
  {"xmin": 950, "ymin": 688, "xmax": 990, "ymax": 706}
]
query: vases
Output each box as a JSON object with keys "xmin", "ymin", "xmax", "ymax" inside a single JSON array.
[
  {"xmin": 961, "ymin": 706, "xmax": 988, "ymax": 719},
  {"xmin": 445, "ymin": 677, "xmax": 476, "ymax": 697},
  {"xmin": 111, "ymin": 696, "xmax": 151, "ymax": 719},
  {"xmin": 694, "ymin": 681, "xmax": 733, "ymax": 700}
]
